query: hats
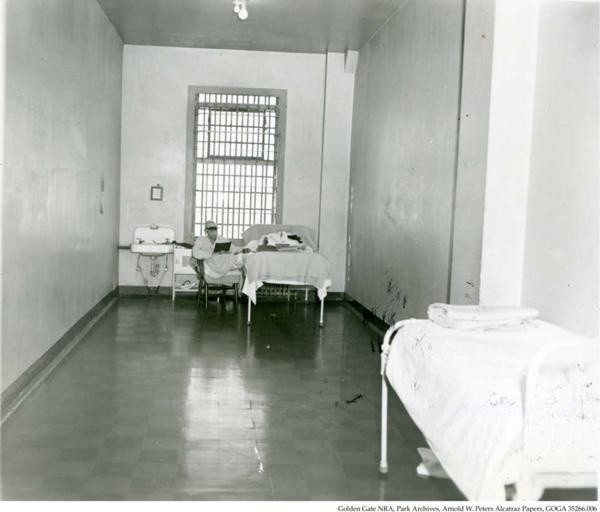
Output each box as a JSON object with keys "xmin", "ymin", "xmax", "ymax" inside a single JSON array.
[{"xmin": 205, "ymin": 221, "xmax": 217, "ymax": 229}]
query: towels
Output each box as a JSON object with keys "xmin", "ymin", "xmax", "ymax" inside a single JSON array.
[{"xmin": 428, "ymin": 302, "xmax": 538, "ymax": 330}]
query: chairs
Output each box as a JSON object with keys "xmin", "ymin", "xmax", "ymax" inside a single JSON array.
[{"xmin": 197, "ymin": 260, "xmax": 238, "ymax": 313}]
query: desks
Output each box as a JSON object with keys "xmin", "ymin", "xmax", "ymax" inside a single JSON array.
[{"xmin": 173, "ymin": 248, "xmax": 220, "ymax": 303}]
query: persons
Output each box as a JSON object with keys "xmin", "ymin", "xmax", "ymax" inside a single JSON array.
[{"xmin": 192, "ymin": 220, "xmax": 242, "ymax": 284}]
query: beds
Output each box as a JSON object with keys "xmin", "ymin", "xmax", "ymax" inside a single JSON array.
[
  {"xmin": 379, "ymin": 318, "xmax": 597, "ymax": 502},
  {"xmin": 241, "ymin": 224, "xmax": 331, "ymax": 326}
]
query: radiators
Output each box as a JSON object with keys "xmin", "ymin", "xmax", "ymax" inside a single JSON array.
[{"xmin": 256, "ymin": 283, "xmax": 297, "ymax": 301}]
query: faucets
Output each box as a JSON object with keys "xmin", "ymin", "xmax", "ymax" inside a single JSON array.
[{"xmin": 152, "ymin": 239, "xmax": 157, "ymax": 244}]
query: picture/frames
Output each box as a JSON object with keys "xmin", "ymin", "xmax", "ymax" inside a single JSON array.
[{"xmin": 151, "ymin": 187, "xmax": 163, "ymax": 200}]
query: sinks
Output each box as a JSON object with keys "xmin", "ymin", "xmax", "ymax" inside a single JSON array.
[{"xmin": 130, "ymin": 241, "xmax": 173, "ymax": 255}]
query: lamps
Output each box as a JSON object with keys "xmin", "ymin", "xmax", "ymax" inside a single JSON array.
[{"xmin": 233, "ymin": 0, "xmax": 248, "ymax": 20}]
